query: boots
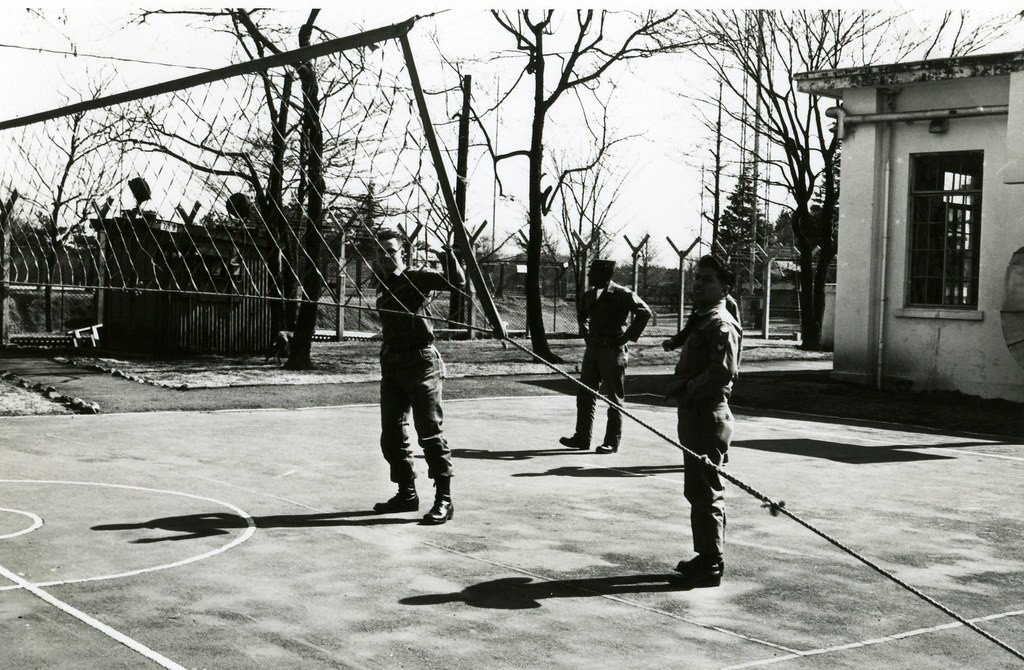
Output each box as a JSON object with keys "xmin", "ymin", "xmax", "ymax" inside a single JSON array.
[
  {"xmin": 424, "ymin": 480, "xmax": 454, "ymax": 523},
  {"xmin": 375, "ymin": 481, "xmax": 420, "ymax": 512}
]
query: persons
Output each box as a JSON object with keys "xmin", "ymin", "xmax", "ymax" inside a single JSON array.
[
  {"xmin": 663, "ymin": 291, "xmax": 741, "ymax": 354},
  {"xmin": 559, "ymin": 259, "xmax": 652, "ymax": 454},
  {"xmin": 664, "ymin": 253, "xmax": 744, "ymax": 588},
  {"xmin": 373, "ymin": 230, "xmax": 467, "ymax": 524}
]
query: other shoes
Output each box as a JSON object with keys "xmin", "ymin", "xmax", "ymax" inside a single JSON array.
[
  {"xmin": 596, "ymin": 444, "xmax": 618, "ymax": 453},
  {"xmin": 679, "ymin": 556, "xmax": 725, "ymax": 574},
  {"xmin": 560, "ymin": 437, "xmax": 589, "ymax": 450}
]
